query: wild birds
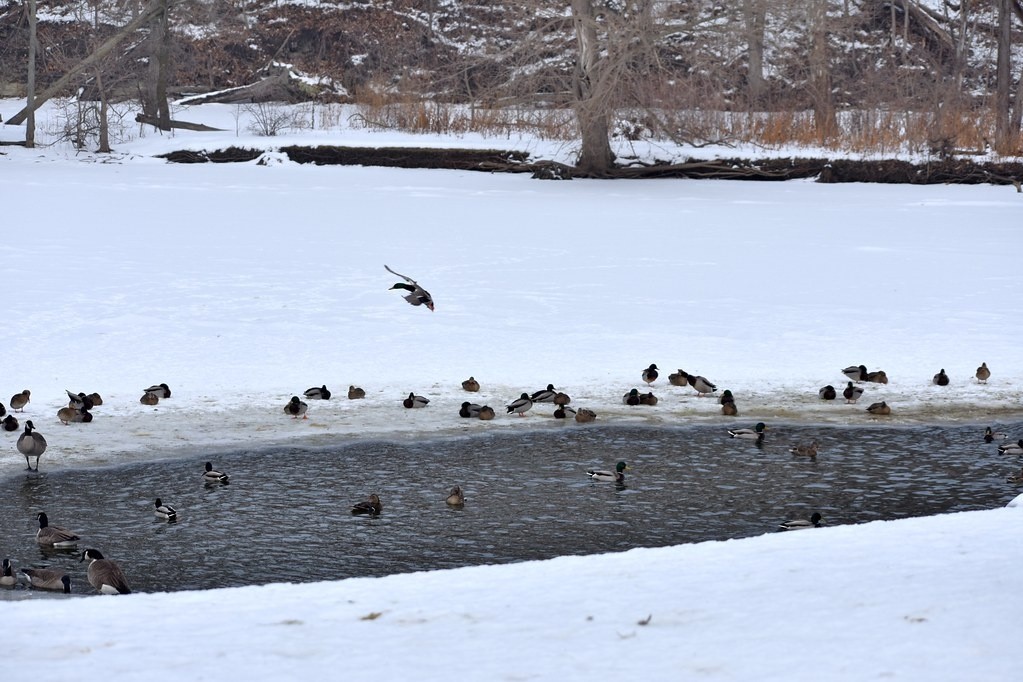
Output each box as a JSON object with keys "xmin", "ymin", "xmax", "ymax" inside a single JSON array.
[
  {"xmin": 0, "ymin": 362, "xmax": 1022, "ymax": 598},
  {"xmin": 383, "ymin": 263, "xmax": 436, "ymax": 311}
]
